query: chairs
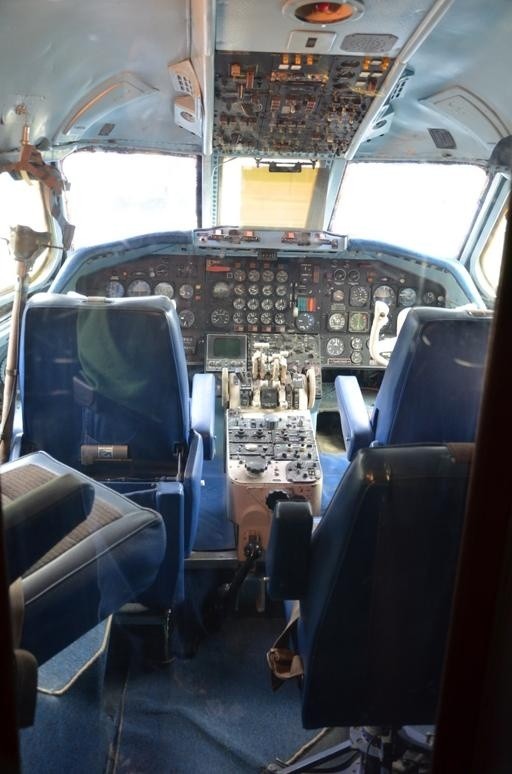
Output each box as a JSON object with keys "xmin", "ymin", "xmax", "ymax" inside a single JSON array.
[
  {"xmin": 263, "ymin": 307, "xmax": 497, "ymax": 730},
  {"xmin": 2, "ymin": 293, "xmax": 214, "ymax": 732}
]
